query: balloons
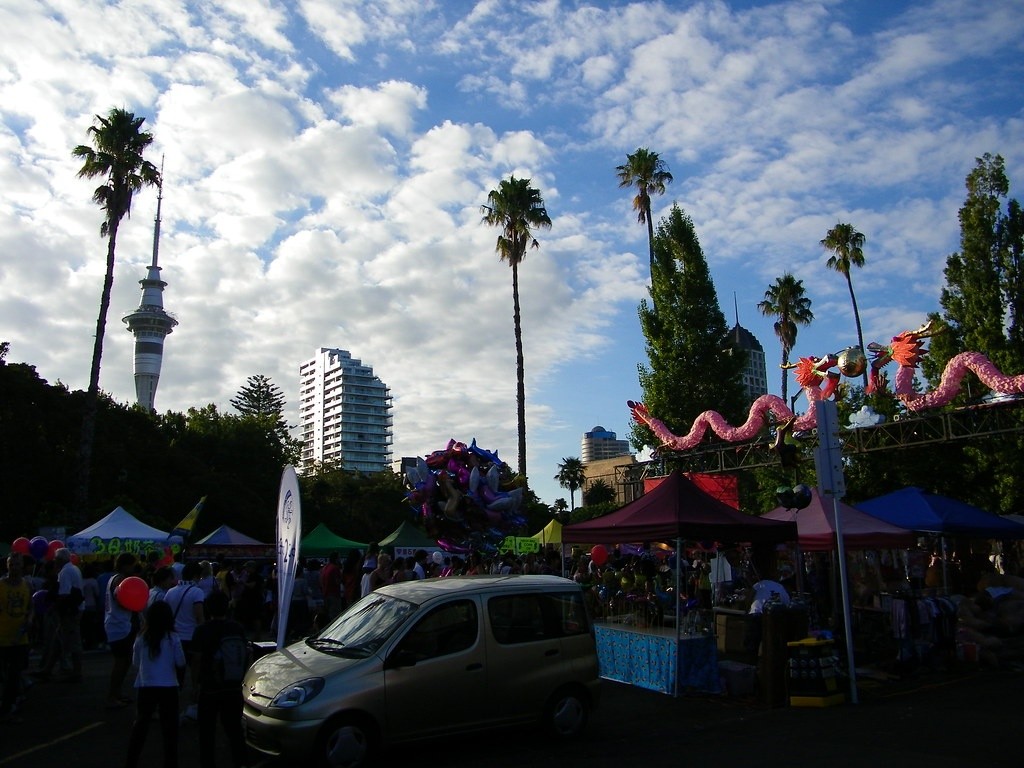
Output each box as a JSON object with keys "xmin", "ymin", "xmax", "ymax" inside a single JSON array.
[
  {"xmin": 776, "ymin": 486, "xmax": 794, "ymax": 509},
  {"xmin": 403, "ymin": 440, "xmax": 527, "ymax": 553},
  {"xmin": 591, "ymin": 545, "xmax": 608, "ymax": 565},
  {"xmin": 595, "ymin": 561, "xmax": 711, "ymax": 609},
  {"xmin": 13, "ymin": 537, "xmax": 65, "ymax": 560},
  {"xmin": 117, "ymin": 577, "xmax": 149, "ymax": 612}
]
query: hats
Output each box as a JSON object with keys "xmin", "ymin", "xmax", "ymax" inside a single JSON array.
[{"xmin": 243, "ymin": 560, "xmax": 256, "ymax": 567}]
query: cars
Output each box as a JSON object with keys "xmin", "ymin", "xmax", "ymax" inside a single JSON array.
[{"xmin": 241, "ymin": 574, "xmax": 603, "ymax": 768}]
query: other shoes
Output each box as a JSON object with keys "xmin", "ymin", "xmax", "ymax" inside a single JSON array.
[
  {"xmin": 19, "ymin": 678, "xmax": 34, "ymax": 689},
  {"xmin": 11, "ymin": 704, "xmax": 24, "ymax": 714},
  {"xmin": 102, "ymin": 696, "xmax": 127, "ymax": 708},
  {"xmin": 16, "ymin": 695, "xmax": 35, "ymax": 705},
  {"xmin": 118, "ymin": 694, "xmax": 135, "ymax": 705},
  {"xmin": 56, "ymin": 673, "xmax": 81, "ymax": 683},
  {"xmin": 1, "ymin": 715, "xmax": 25, "ymax": 725}
]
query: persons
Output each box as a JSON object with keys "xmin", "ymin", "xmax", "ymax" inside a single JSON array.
[
  {"xmin": 1, "ymin": 549, "xmax": 341, "ymax": 768},
  {"xmin": 543, "ymin": 547, "xmax": 588, "ymax": 607},
  {"xmin": 344, "ymin": 551, "xmax": 539, "ymax": 615}
]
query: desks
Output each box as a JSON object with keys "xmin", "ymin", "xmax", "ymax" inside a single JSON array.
[
  {"xmin": 594, "ymin": 620, "xmax": 726, "ymax": 702},
  {"xmin": 713, "ymin": 605, "xmax": 745, "ymax": 653}
]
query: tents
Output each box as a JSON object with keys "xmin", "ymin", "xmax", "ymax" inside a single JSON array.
[
  {"xmin": 562, "ymin": 469, "xmax": 803, "ymax": 696},
  {"xmin": 300, "ymin": 523, "xmax": 370, "ymax": 563},
  {"xmin": 66, "ymin": 506, "xmax": 177, "ymax": 559},
  {"xmin": 759, "ymin": 487, "xmax": 913, "ymax": 639},
  {"xmin": 852, "ymin": 488, "xmax": 1024, "ymax": 637},
  {"xmin": 192, "ymin": 524, "xmax": 276, "ymax": 556},
  {"xmin": 529, "ymin": 518, "xmax": 561, "ymax": 544},
  {"xmin": 378, "ymin": 521, "xmax": 436, "ymax": 546}
]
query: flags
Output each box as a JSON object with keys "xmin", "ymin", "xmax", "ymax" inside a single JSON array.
[{"xmin": 166, "ymin": 499, "xmax": 206, "ymax": 541}]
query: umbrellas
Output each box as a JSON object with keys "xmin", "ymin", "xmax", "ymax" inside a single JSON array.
[{"xmin": 792, "ymin": 484, "xmax": 812, "ymax": 510}]
